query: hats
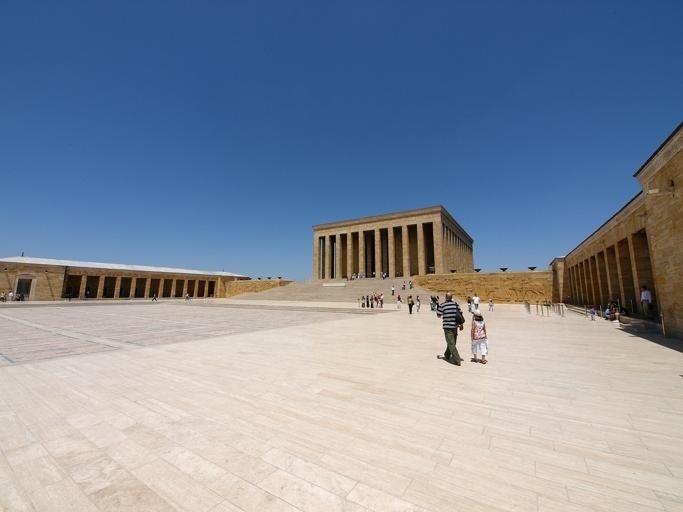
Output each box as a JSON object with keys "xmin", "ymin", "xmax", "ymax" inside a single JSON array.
[{"xmin": 472, "ymin": 310, "xmax": 482, "ymax": 317}]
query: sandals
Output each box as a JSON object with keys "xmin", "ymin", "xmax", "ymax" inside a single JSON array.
[{"xmin": 472, "ymin": 358, "xmax": 486, "ymax": 364}]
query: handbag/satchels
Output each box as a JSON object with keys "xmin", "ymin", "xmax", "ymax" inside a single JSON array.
[
  {"xmin": 455, "ymin": 302, "xmax": 465, "ymax": 325},
  {"xmin": 411, "ymin": 299, "xmax": 414, "ymax": 305}
]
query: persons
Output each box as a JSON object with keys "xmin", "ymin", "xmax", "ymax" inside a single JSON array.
[
  {"xmin": 183, "ymin": 292, "xmax": 191, "ymax": 304},
  {"xmin": 602, "ymin": 301, "xmax": 627, "ymax": 321},
  {"xmin": 589, "ymin": 305, "xmax": 596, "ymax": 321},
  {"xmin": 435, "ymin": 292, "xmax": 495, "ymax": 366},
  {"xmin": 151, "ymin": 287, "xmax": 157, "ymax": 301},
  {"xmin": 0, "ymin": 290, "xmax": 24, "ymax": 302},
  {"xmin": 639, "ymin": 286, "xmax": 652, "ymax": 320},
  {"xmin": 356, "ymin": 281, "xmax": 441, "ymax": 315},
  {"xmin": 352, "ymin": 271, "xmax": 387, "ymax": 280}
]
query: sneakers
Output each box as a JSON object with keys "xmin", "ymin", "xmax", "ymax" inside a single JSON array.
[{"xmin": 444, "ymin": 353, "xmax": 461, "ymax": 366}]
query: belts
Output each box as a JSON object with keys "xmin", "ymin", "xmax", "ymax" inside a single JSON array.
[{"xmin": 642, "ymin": 299, "xmax": 649, "ymax": 301}]
